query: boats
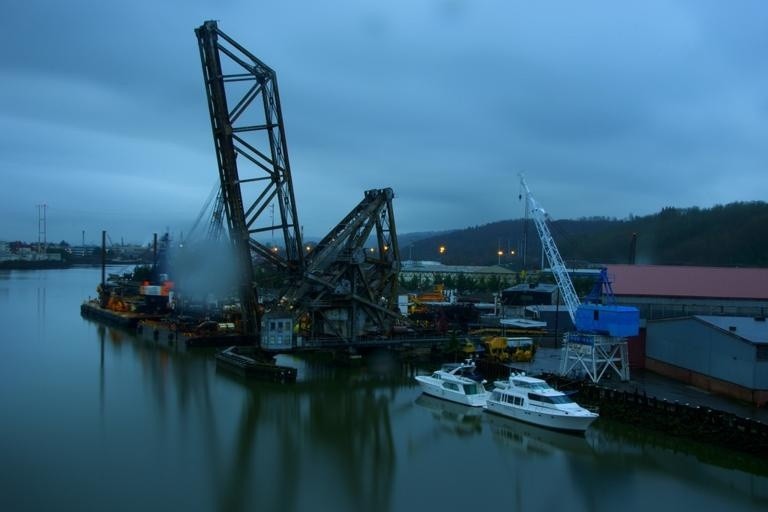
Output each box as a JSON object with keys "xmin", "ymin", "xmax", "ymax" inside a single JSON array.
[
  {"xmin": 486, "ymin": 372, "xmax": 598, "ymax": 436},
  {"xmin": 412, "ymin": 371, "xmax": 490, "ymax": 408}
]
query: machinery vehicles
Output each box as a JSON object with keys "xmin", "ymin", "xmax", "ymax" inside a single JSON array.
[{"xmin": 458, "ymin": 337, "xmax": 536, "ymax": 362}]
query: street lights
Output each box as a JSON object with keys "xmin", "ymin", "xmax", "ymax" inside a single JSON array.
[{"xmin": 498, "ymin": 251, "xmax": 503, "ymax": 264}]
